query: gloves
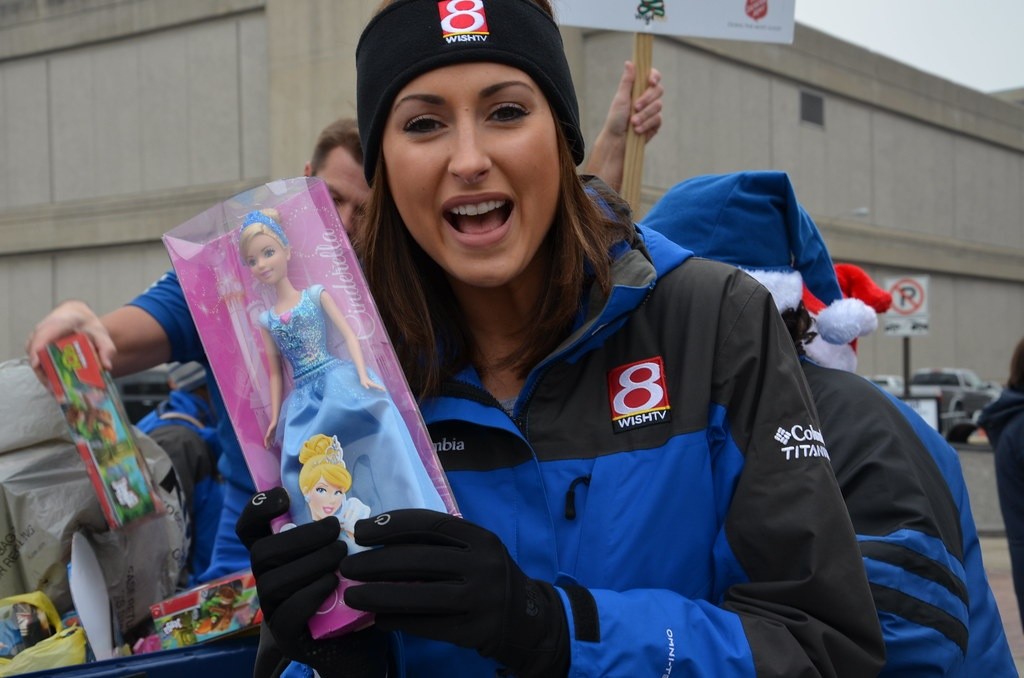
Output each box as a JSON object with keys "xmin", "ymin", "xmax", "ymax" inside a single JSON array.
[
  {"xmin": 339, "ymin": 508, "xmax": 571, "ymax": 677},
  {"xmin": 236, "ymin": 487, "xmax": 399, "ymax": 678}
]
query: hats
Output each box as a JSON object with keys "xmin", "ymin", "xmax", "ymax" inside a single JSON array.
[
  {"xmin": 355, "ymin": 0, "xmax": 585, "ymax": 189},
  {"xmin": 637, "ymin": 170, "xmax": 859, "ymax": 345},
  {"xmin": 797, "ymin": 263, "xmax": 894, "ymax": 373}
]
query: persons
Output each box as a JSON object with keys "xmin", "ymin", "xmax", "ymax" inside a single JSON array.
[
  {"xmin": 238, "ymin": 209, "xmax": 447, "ymax": 555},
  {"xmin": 977, "ymin": 339, "xmax": 1024, "ymax": 628},
  {"xmin": 235, "ymin": 0, "xmax": 887, "ymax": 678},
  {"xmin": 28, "ymin": 61, "xmax": 665, "ymax": 589},
  {"xmin": 639, "ymin": 170, "xmax": 1018, "ymax": 678}
]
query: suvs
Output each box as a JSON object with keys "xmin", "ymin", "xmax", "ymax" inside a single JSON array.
[{"xmin": 862, "ymin": 367, "xmax": 1004, "ymax": 442}]
